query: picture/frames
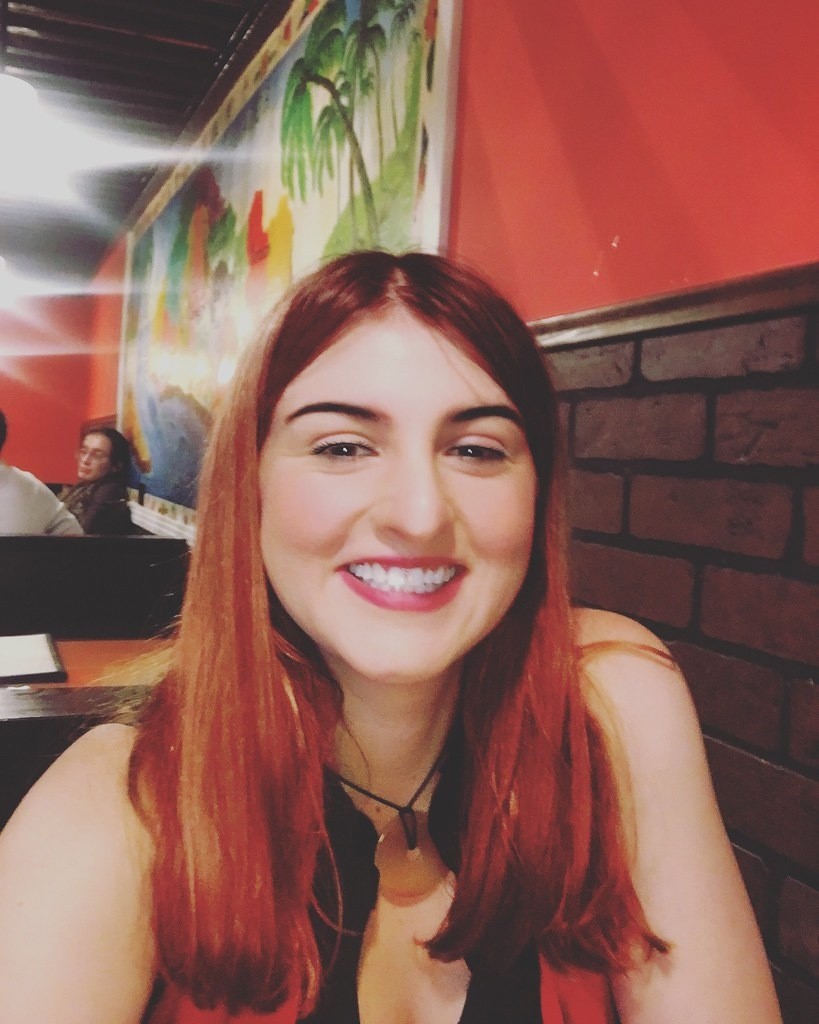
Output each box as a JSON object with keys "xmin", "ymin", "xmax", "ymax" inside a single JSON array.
[{"xmin": 112, "ymin": 0, "xmax": 462, "ymax": 554}]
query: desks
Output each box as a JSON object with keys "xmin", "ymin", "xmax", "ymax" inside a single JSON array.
[{"xmin": 0, "ymin": 630, "xmax": 169, "ymax": 818}]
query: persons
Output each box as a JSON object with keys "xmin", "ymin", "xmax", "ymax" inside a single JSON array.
[
  {"xmin": 1, "ymin": 251, "xmax": 784, "ymax": 1024},
  {"xmin": 0, "ymin": 411, "xmax": 86, "ymax": 535},
  {"xmin": 56, "ymin": 427, "xmax": 127, "ymax": 535}
]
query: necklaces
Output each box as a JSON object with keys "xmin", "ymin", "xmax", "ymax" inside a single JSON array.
[{"xmin": 323, "ymin": 722, "xmax": 450, "ymax": 899}]
